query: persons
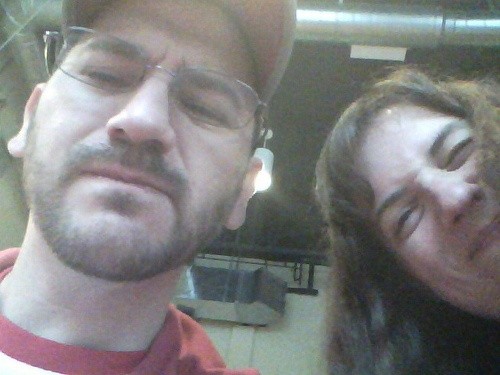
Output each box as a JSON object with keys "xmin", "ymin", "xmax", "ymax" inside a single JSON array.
[
  {"xmin": 308, "ymin": 61, "xmax": 499, "ymax": 375},
  {"xmin": 0, "ymin": 0, "xmax": 299, "ymax": 375}
]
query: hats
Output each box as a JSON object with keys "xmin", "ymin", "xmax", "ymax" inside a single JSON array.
[{"xmin": 62, "ymin": 0, "xmax": 297, "ymax": 102}]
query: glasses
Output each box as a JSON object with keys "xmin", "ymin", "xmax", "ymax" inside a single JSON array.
[{"xmin": 42, "ymin": 27, "xmax": 267, "ymax": 133}]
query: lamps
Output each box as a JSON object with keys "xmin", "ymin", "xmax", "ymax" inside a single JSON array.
[{"xmin": 251, "ymin": 127, "xmax": 274, "ymax": 195}]
